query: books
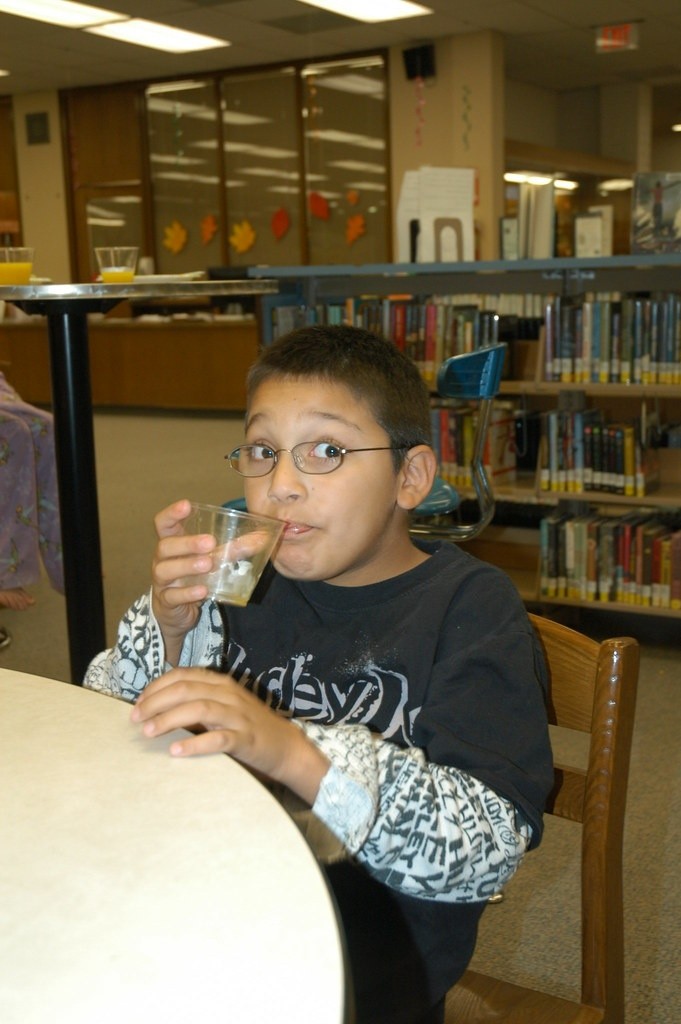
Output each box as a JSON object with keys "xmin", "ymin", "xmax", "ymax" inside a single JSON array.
[
  {"xmin": 539, "ymin": 512, "xmax": 681, "ymax": 609},
  {"xmin": 428, "ymin": 396, "xmax": 517, "ymax": 489},
  {"xmin": 538, "ymin": 406, "xmax": 661, "ymax": 499},
  {"xmin": 536, "ymin": 294, "xmax": 681, "ymax": 386},
  {"xmin": 273, "ymin": 296, "xmax": 502, "ymax": 394}
]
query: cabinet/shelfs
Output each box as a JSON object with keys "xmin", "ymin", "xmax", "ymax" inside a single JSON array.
[{"xmin": 250, "ymin": 251, "xmax": 680, "ymax": 648}]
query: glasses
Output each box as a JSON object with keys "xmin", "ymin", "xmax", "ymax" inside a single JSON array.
[{"xmin": 224, "ymin": 439, "xmax": 405, "ymax": 475}]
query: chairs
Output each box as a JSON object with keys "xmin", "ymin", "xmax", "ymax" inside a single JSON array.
[
  {"xmin": 411, "ymin": 342, "xmax": 508, "ymax": 540},
  {"xmin": 409, "ymin": 614, "xmax": 641, "ymax": 1024}
]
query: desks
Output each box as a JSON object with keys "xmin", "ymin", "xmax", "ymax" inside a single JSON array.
[
  {"xmin": 2, "ymin": 669, "xmax": 358, "ymax": 1024},
  {"xmin": 0, "ymin": 278, "xmax": 277, "ymax": 686},
  {"xmin": 1, "ymin": 311, "xmax": 260, "ymax": 412}
]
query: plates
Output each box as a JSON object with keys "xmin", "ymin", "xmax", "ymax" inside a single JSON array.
[
  {"xmin": 136, "ymin": 270, "xmax": 205, "ymax": 281},
  {"xmin": 31, "ymin": 277, "xmax": 52, "ymax": 285}
]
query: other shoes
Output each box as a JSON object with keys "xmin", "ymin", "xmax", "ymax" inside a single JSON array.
[{"xmin": 0, "ymin": 631, "xmax": 11, "ymax": 648}]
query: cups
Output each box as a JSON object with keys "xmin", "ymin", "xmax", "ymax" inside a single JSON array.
[
  {"xmin": 94, "ymin": 246, "xmax": 138, "ymax": 283},
  {"xmin": 0, "ymin": 248, "xmax": 34, "ymax": 285},
  {"xmin": 180, "ymin": 503, "xmax": 287, "ymax": 607}
]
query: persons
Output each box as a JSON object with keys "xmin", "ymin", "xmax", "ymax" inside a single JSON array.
[
  {"xmin": 81, "ymin": 325, "xmax": 555, "ymax": 1024},
  {"xmin": 0, "ymin": 370, "xmax": 65, "ymax": 610}
]
